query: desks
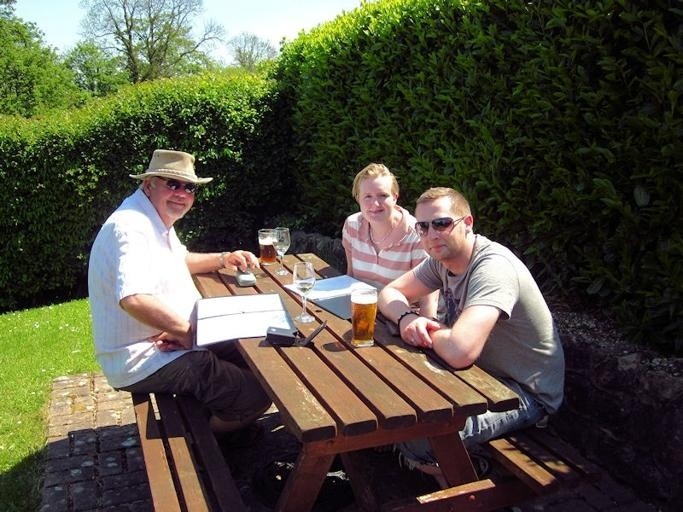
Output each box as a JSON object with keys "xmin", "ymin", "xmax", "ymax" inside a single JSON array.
[{"xmin": 191, "ymin": 253, "xmax": 521, "ymax": 512}]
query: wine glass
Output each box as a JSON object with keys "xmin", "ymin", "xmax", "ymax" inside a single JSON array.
[
  {"xmin": 293, "ymin": 261, "xmax": 317, "ymax": 324},
  {"xmin": 271, "ymin": 227, "xmax": 292, "ymax": 276}
]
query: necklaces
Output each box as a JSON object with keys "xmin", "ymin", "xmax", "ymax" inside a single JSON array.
[{"xmin": 370, "ymin": 227, "xmax": 394, "ymax": 244}]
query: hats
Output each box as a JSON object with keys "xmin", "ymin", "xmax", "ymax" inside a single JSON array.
[{"xmin": 127, "ymin": 148, "xmax": 213, "ymax": 186}]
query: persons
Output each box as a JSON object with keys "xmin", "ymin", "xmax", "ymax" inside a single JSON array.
[
  {"xmin": 341, "ymin": 163, "xmax": 447, "ymax": 320},
  {"xmin": 88, "ymin": 149, "xmax": 274, "ymax": 470},
  {"xmin": 375, "ymin": 186, "xmax": 567, "ymax": 489}
]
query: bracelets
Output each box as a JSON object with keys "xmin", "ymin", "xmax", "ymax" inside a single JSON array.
[
  {"xmin": 396, "ymin": 310, "xmax": 420, "ymax": 328},
  {"xmin": 220, "ymin": 251, "xmax": 231, "ymax": 268}
]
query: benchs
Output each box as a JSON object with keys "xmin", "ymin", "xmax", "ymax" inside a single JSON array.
[
  {"xmin": 485, "ymin": 428, "xmax": 610, "ymax": 512},
  {"xmin": 131, "ymin": 390, "xmax": 249, "ymax": 512}
]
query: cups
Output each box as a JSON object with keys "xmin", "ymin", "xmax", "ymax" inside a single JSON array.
[
  {"xmin": 348, "ymin": 288, "xmax": 379, "ymax": 349},
  {"xmin": 257, "ymin": 228, "xmax": 278, "ymax": 267}
]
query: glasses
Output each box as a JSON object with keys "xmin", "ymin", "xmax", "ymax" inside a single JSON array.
[
  {"xmin": 154, "ymin": 175, "xmax": 199, "ymax": 193},
  {"xmin": 413, "ymin": 215, "xmax": 466, "ymax": 238}
]
